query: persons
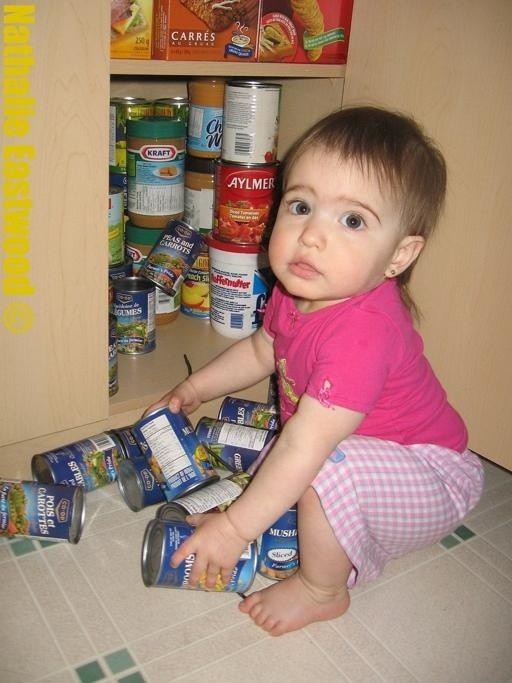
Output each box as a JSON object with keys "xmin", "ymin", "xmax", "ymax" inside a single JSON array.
[{"xmin": 141, "ymin": 100, "xmax": 485, "ymax": 638}]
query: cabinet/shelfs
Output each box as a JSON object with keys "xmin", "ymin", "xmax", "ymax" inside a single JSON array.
[{"xmin": 0, "ymin": 0, "xmax": 357, "ymax": 483}]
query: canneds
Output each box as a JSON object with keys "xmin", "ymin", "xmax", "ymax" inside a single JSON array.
[
  {"xmin": 2, "ymin": 478, "xmax": 85, "ymax": 544},
  {"xmin": 32, "ymin": 396, "xmax": 300, "ymax": 580},
  {"xmin": 207, "ymin": 78, "xmax": 285, "ymax": 343},
  {"xmin": 110, "ymin": 77, "xmax": 225, "ymax": 401},
  {"xmin": 141, "ymin": 519, "xmax": 258, "ymax": 593}
]
